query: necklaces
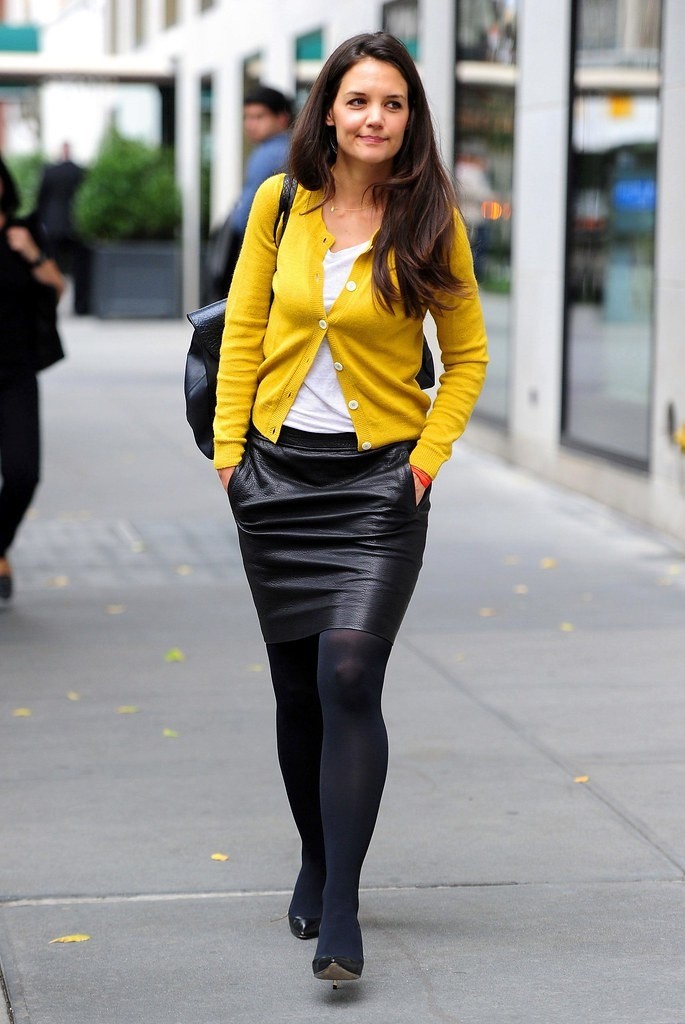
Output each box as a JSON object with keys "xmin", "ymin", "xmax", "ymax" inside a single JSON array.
[{"xmin": 326, "ymin": 163, "xmax": 382, "ymax": 212}]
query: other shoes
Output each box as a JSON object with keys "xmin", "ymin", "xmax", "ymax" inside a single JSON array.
[{"xmin": 0, "ymin": 574, "xmax": 14, "ymax": 600}]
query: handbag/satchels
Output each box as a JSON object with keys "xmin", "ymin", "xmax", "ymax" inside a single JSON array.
[
  {"xmin": 0, "ymin": 212, "xmax": 66, "ymax": 372},
  {"xmin": 184, "ymin": 297, "xmax": 227, "ymax": 460}
]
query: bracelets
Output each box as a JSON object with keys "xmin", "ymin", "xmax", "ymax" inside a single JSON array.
[{"xmin": 409, "ymin": 465, "xmax": 432, "ymax": 490}]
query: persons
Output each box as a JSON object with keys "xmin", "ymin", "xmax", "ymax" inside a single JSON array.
[
  {"xmin": 212, "ymin": 30, "xmax": 490, "ymax": 989},
  {"xmin": 212, "ymin": 87, "xmax": 299, "ymax": 298},
  {"xmin": 0, "ymin": 156, "xmax": 67, "ymax": 600},
  {"xmin": 36, "ymin": 140, "xmax": 90, "ymax": 274}
]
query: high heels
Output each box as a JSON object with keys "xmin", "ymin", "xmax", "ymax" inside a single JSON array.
[
  {"xmin": 287, "ymin": 911, "xmax": 323, "ymax": 939},
  {"xmin": 312, "ymin": 926, "xmax": 364, "ymax": 990}
]
